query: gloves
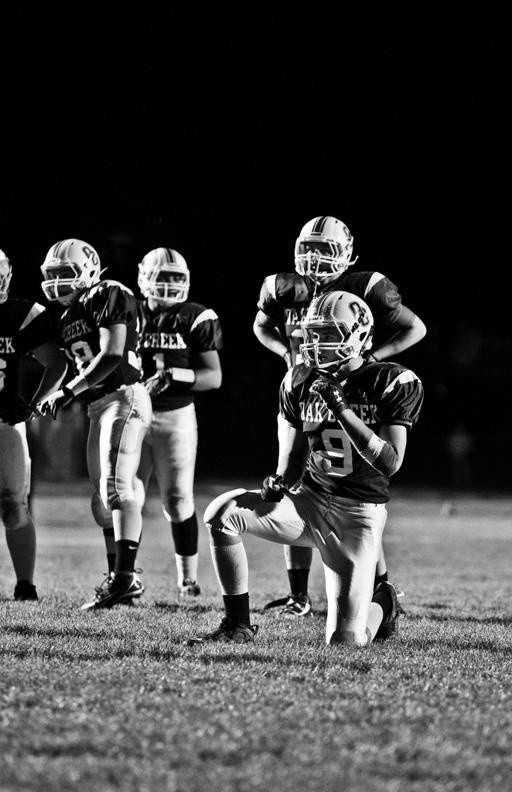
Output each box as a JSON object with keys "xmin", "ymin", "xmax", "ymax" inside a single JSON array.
[
  {"xmin": 38, "ymin": 387, "xmax": 74, "ymax": 420},
  {"xmin": 145, "ymin": 368, "xmax": 171, "ymax": 397},
  {"xmin": 308, "ymin": 369, "xmax": 351, "ymax": 419},
  {"xmin": 260, "ymin": 474, "xmax": 284, "ymax": 503}
]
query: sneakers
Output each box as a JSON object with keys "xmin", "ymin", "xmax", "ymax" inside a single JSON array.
[
  {"xmin": 278, "ymin": 594, "xmax": 313, "ymax": 622},
  {"xmin": 371, "ymin": 581, "xmax": 400, "ymax": 644},
  {"xmin": 14, "ymin": 582, "xmax": 39, "ymax": 606},
  {"xmin": 80, "ymin": 571, "xmax": 145, "ymax": 612},
  {"xmin": 182, "ymin": 616, "xmax": 259, "ymax": 646},
  {"xmin": 176, "ymin": 582, "xmax": 207, "ymax": 613}
]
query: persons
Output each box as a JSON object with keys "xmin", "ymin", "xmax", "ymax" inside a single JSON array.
[
  {"xmin": 0, "ymin": 248, "xmax": 69, "ymax": 604},
  {"xmin": 106, "ymin": 246, "xmax": 225, "ymax": 597},
  {"xmin": 252, "ymin": 214, "xmax": 429, "ymax": 614},
  {"xmin": 27, "ymin": 236, "xmax": 155, "ymax": 611},
  {"xmin": 131, "ymin": 242, "xmax": 225, "ymax": 602},
  {"xmin": 182, "ymin": 289, "xmax": 427, "ymax": 651}
]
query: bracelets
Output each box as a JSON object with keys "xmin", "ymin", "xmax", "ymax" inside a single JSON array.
[
  {"xmin": 359, "ymin": 432, "xmax": 396, "ymax": 477},
  {"xmin": 63, "ymin": 375, "xmax": 90, "ymax": 397},
  {"xmin": 165, "ymin": 365, "xmax": 198, "ymax": 389}
]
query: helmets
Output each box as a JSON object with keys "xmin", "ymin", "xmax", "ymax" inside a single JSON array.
[
  {"xmin": 294, "ymin": 216, "xmax": 354, "ymax": 282},
  {"xmin": 137, "ymin": 247, "xmax": 191, "ymax": 307},
  {"xmin": 40, "ymin": 238, "xmax": 101, "ymax": 302},
  {"xmin": 0, "ymin": 249, "xmax": 13, "ymax": 305},
  {"xmin": 298, "ymin": 290, "xmax": 374, "ymax": 369}
]
